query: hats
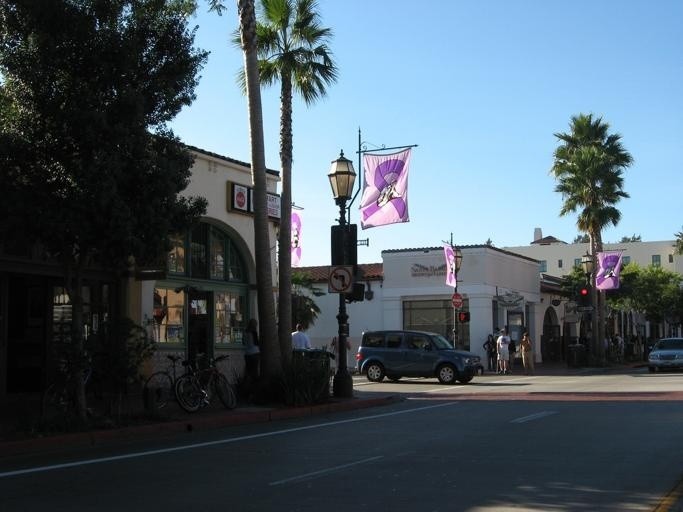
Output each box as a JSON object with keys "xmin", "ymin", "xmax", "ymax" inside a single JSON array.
[{"xmin": 499, "ymin": 329, "xmax": 504, "ymax": 332}]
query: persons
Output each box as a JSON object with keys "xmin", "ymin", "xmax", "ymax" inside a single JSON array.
[
  {"xmin": 289, "ymin": 322, "xmax": 311, "ymax": 353},
  {"xmin": 482, "ymin": 333, "xmax": 496, "ymax": 370},
  {"xmin": 496, "ymin": 327, "xmax": 510, "ymax": 374},
  {"xmin": 516, "ymin": 329, "xmax": 535, "ymax": 376},
  {"xmin": 598, "ymin": 331, "xmax": 650, "ymax": 365},
  {"xmin": 507, "ymin": 338, "xmax": 517, "ymax": 372},
  {"xmin": 242, "ymin": 319, "xmax": 258, "ymax": 370}
]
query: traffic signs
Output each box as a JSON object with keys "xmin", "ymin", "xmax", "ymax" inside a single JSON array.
[
  {"xmin": 327, "ymin": 266, "xmax": 354, "ymax": 293},
  {"xmin": 577, "ymin": 305, "xmax": 595, "ymax": 312}
]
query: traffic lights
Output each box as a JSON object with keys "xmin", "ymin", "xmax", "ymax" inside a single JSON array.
[
  {"xmin": 458, "ymin": 311, "xmax": 469, "ymax": 324},
  {"xmin": 578, "ymin": 286, "xmax": 589, "ymax": 298}
]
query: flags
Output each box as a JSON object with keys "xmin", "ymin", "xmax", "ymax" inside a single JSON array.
[
  {"xmin": 289, "ymin": 210, "xmax": 301, "ymax": 268},
  {"xmin": 594, "ymin": 251, "xmax": 624, "ymax": 290},
  {"xmin": 445, "ymin": 243, "xmax": 458, "ymax": 288},
  {"xmin": 356, "ymin": 147, "xmax": 413, "ymax": 230}
]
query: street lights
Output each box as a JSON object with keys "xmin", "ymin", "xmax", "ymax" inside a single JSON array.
[
  {"xmin": 327, "ymin": 148, "xmax": 356, "ymax": 397},
  {"xmin": 451, "ymin": 245, "xmax": 462, "ymax": 349},
  {"xmin": 580, "ymin": 251, "xmax": 594, "ymax": 368}
]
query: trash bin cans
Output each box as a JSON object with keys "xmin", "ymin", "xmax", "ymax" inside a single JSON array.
[
  {"xmin": 293, "ymin": 348, "xmax": 331, "ymax": 403},
  {"xmin": 568, "ymin": 344, "xmax": 585, "ymax": 368}
]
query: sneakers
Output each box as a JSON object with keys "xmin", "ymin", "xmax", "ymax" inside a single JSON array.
[{"xmin": 498, "ymin": 370, "xmax": 507, "ymax": 375}]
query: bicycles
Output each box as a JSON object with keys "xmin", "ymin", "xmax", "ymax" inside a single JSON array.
[
  {"xmin": 143, "ymin": 352, "xmax": 237, "ymax": 412},
  {"xmin": 41, "ymin": 357, "xmax": 109, "ymax": 423}
]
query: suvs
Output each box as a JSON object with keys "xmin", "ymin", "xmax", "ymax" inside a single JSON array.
[{"xmin": 356, "ymin": 329, "xmax": 480, "ymax": 384}]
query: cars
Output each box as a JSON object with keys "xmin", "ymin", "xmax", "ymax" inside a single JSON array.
[{"xmin": 647, "ymin": 337, "xmax": 682, "ymax": 372}]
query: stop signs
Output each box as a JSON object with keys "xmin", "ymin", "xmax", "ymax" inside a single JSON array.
[{"xmin": 451, "ymin": 293, "xmax": 463, "ymax": 308}]
